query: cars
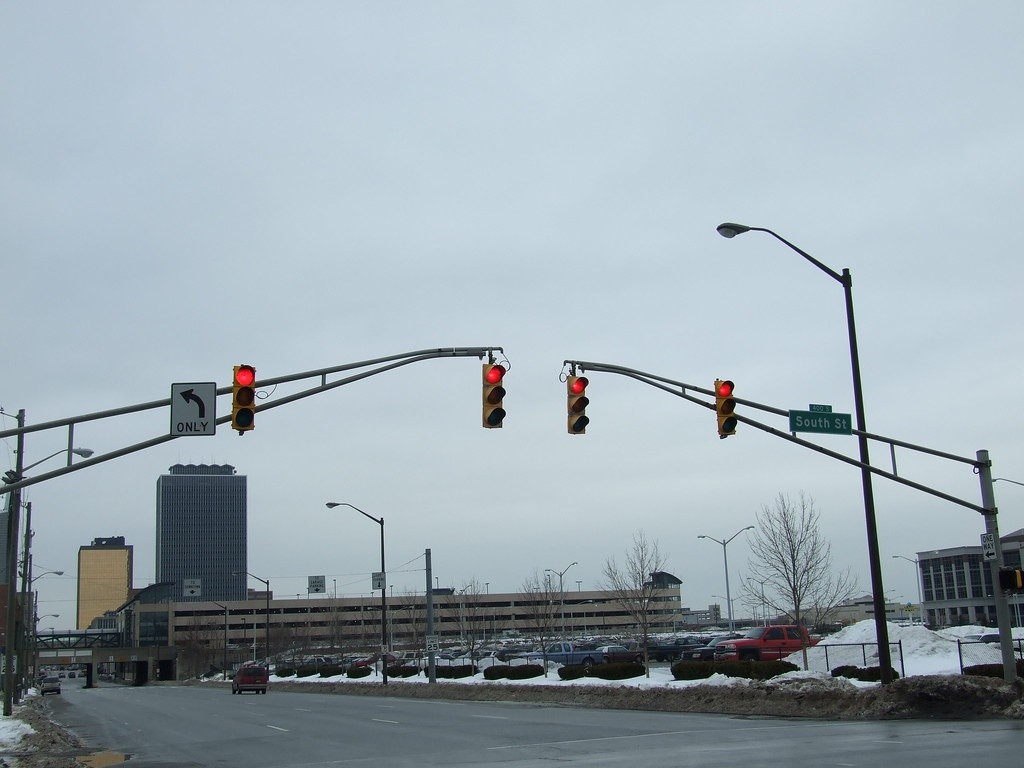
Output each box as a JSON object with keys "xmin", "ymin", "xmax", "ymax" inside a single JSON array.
[
  {"xmin": 40, "ymin": 665, "xmax": 58, "ymax": 672},
  {"xmin": 232, "ymin": 667, "xmax": 268, "ymax": 694},
  {"xmin": 40, "ymin": 676, "xmax": 62, "ymax": 695},
  {"xmin": 284, "ymin": 633, "xmax": 751, "ymax": 667},
  {"xmin": 59, "ymin": 671, "xmax": 85, "ymax": 678}
]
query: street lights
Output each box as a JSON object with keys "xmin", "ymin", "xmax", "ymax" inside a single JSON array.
[
  {"xmin": 32, "ymin": 591, "xmax": 60, "ymax": 687},
  {"xmin": 746, "ymin": 573, "xmax": 779, "ymax": 627},
  {"xmin": 715, "ymin": 222, "xmax": 893, "ymax": 684},
  {"xmin": 325, "ymin": 501, "xmax": 388, "ymax": 684},
  {"xmin": 893, "ymin": 555, "xmax": 924, "ymax": 626},
  {"xmin": 2, "ymin": 408, "xmax": 95, "ymax": 716},
  {"xmin": 545, "ymin": 561, "xmax": 593, "ymax": 641},
  {"xmin": 367, "ymin": 605, "xmax": 414, "ymax": 654},
  {"xmin": 231, "ymin": 571, "xmax": 270, "ymax": 669},
  {"xmin": 25, "ymin": 554, "xmax": 65, "ymax": 695},
  {"xmin": 698, "ymin": 525, "xmax": 756, "ymax": 637},
  {"xmin": 205, "ymin": 599, "xmax": 227, "ymax": 680},
  {"xmin": 332, "ymin": 579, "xmax": 336, "ymax": 600}
]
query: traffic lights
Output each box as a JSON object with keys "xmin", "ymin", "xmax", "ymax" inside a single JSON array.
[
  {"xmin": 482, "ymin": 362, "xmax": 507, "ymax": 428},
  {"xmin": 566, "ymin": 375, "xmax": 589, "ymax": 435},
  {"xmin": 232, "ymin": 363, "xmax": 256, "ymax": 433},
  {"xmin": 713, "ymin": 378, "xmax": 738, "ymax": 436},
  {"xmin": 1000, "ymin": 570, "xmax": 1016, "ymax": 592}
]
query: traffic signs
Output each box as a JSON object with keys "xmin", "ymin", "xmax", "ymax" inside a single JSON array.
[
  {"xmin": 372, "ymin": 572, "xmax": 386, "ymax": 589},
  {"xmin": 308, "ymin": 576, "xmax": 325, "ymax": 594},
  {"xmin": 183, "ymin": 578, "xmax": 202, "ymax": 597},
  {"xmin": 980, "ymin": 533, "xmax": 997, "ymax": 561},
  {"xmin": 170, "ymin": 382, "xmax": 216, "ymax": 437}
]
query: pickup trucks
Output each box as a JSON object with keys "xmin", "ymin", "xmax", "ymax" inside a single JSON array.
[{"xmin": 713, "ymin": 624, "xmax": 822, "ymax": 664}]
